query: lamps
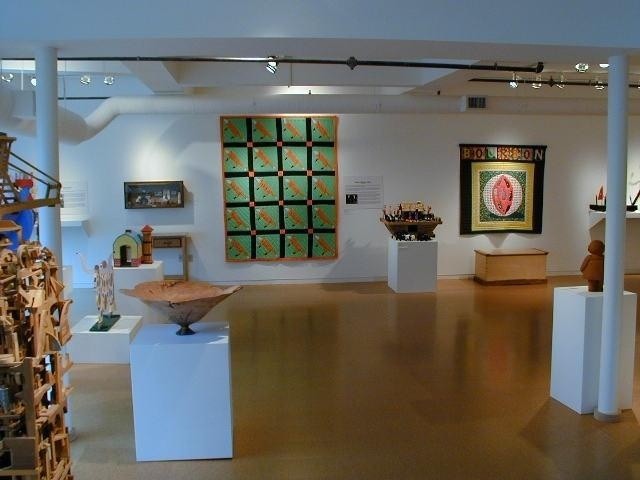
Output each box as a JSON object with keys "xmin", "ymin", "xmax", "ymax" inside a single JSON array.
[
  {"xmin": 509, "ymin": 71, "xmax": 606, "ymax": 92},
  {"xmin": 1, "ymin": 69, "xmax": 116, "ymax": 89},
  {"xmin": 265, "ymin": 53, "xmax": 280, "ymax": 74}
]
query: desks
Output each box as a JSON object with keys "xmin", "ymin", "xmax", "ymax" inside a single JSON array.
[
  {"xmin": 138, "ymin": 233, "xmax": 191, "ymax": 282},
  {"xmin": 472, "ymin": 247, "xmax": 549, "ymax": 286}
]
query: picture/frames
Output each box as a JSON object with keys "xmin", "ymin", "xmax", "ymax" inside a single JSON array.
[{"xmin": 123, "ymin": 180, "xmax": 184, "ymax": 210}]
